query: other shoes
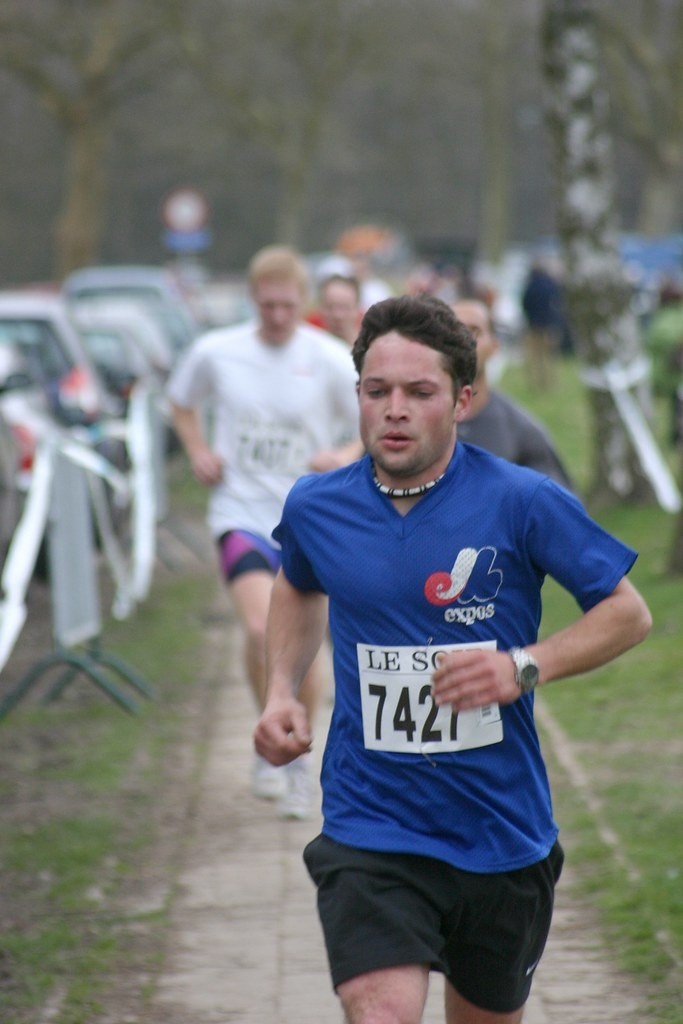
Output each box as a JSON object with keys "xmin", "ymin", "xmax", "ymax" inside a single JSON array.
[
  {"xmin": 246, "ymin": 749, "xmax": 291, "ymax": 801},
  {"xmin": 273, "ymin": 768, "xmax": 308, "ymax": 822}
]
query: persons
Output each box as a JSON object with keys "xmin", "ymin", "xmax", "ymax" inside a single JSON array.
[
  {"xmin": 428, "ymin": 280, "xmax": 573, "ymax": 490},
  {"xmin": 166, "ymin": 245, "xmax": 366, "ymax": 825},
  {"xmin": 255, "ymin": 295, "xmax": 653, "ymax": 1023},
  {"xmin": 315, "ymin": 276, "xmax": 362, "ymax": 342},
  {"xmin": 522, "ymin": 257, "xmax": 561, "ymax": 390}
]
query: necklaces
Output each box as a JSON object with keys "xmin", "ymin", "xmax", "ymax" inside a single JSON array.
[{"xmin": 373, "ymin": 471, "xmax": 446, "ymax": 497}]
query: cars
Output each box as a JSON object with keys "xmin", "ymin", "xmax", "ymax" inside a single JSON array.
[{"xmin": 0, "ymin": 256, "xmax": 251, "ymax": 575}]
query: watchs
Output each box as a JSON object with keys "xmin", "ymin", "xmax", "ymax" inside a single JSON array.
[{"xmin": 508, "ymin": 646, "xmax": 538, "ymax": 692}]
